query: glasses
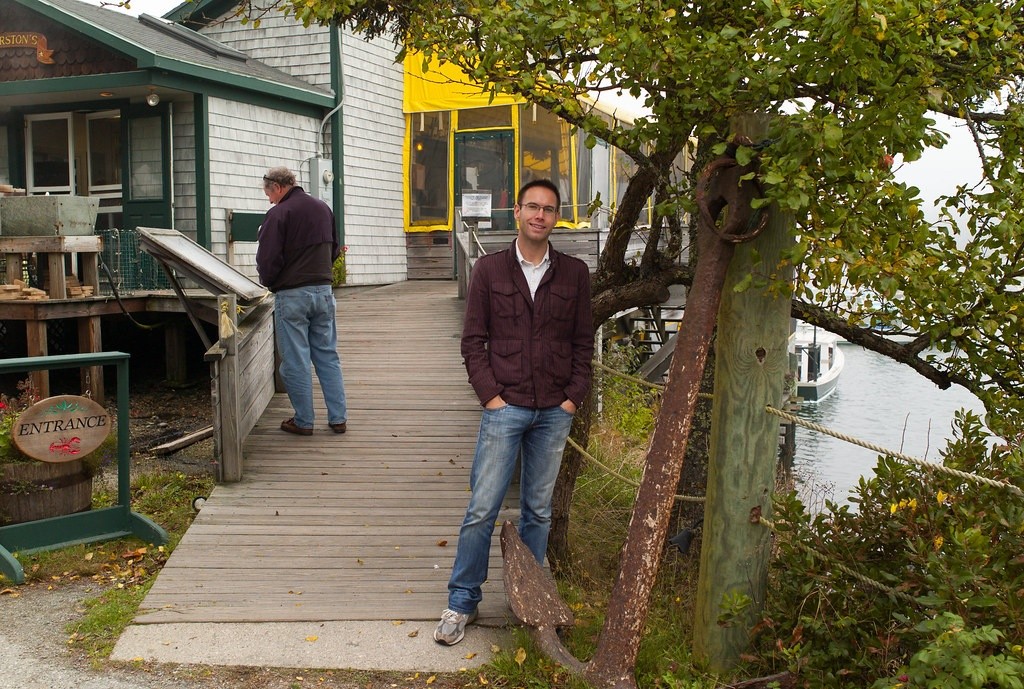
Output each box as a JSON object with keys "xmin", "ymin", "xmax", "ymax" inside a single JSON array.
[
  {"xmin": 263, "ymin": 175, "xmax": 276, "ymax": 182},
  {"xmin": 519, "ymin": 203, "xmax": 557, "ymax": 215}
]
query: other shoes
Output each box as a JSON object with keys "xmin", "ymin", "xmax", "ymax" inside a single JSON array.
[
  {"xmin": 281, "ymin": 417, "xmax": 314, "ymax": 436},
  {"xmin": 328, "ymin": 420, "xmax": 346, "ymax": 433}
]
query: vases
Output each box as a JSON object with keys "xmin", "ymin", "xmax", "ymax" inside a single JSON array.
[{"xmin": 0, "ymin": 459, "xmax": 92, "ymax": 523}]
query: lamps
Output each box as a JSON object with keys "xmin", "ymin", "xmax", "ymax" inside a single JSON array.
[{"xmin": 146, "ymin": 94, "xmax": 159, "ymax": 107}]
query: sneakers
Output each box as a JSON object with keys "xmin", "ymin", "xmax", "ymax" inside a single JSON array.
[{"xmin": 434, "ymin": 607, "xmax": 479, "ymax": 646}]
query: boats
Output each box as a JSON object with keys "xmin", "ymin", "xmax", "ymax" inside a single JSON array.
[{"xmin": 788, "ymin": 311, "xmax": 920, "ymax": 405}]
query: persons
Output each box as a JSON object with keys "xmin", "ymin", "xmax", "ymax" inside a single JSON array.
[
  {"xmin": 433, "ymin": 179, "xmax": 595, "ymax": 647},
  {"xmin": 256, "ymin": 166, "xmax": 347, "ymax": 435}
]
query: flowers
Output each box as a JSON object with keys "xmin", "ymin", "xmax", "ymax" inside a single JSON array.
[{"xmin": 0, "ymin": 372, "xmax": 119, "ymax": 524}]
